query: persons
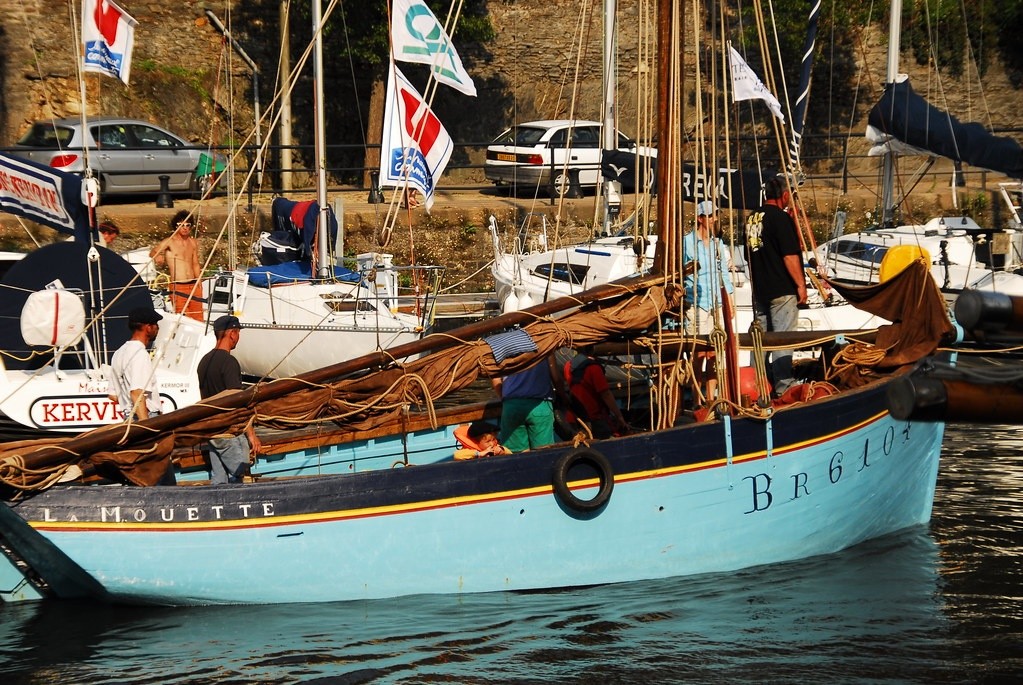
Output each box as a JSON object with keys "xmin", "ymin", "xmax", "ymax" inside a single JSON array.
[
  {"xmin": 454, "ymin": 348, "xmax": 632, "ymax": 461},
  {"xmin": 149, "ymin": 210, "xmax": 204, "ymax": 322},
  {"xmin": 682, "ymin": 201, "xmax": 734, "ymax": 336},
  {"xmin": 744, "ymin": 176, "xmax": 808, "ymax": 398},
  {"xmin": 63, "ymin": 221, "xmax": 119, "ymax": 248},
  {"xmin": 111, "ymin": 308, "xmax": 177, "ymax": 487},
  {"xmin": 197, "ymin": 316, "xmax": 262, "ymax": 484}
]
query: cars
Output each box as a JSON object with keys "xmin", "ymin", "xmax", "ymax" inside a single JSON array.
[
  {"xmin": 0, "ymin": 114, "xmax": 227, "ymax": 209},
  {"xmin": 484, "ymin": 119, "xmax": 658, "ymax": 198}
]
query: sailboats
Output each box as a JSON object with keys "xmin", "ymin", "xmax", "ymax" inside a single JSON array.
[{"xmin": 0, "ymin": 0, "xmax": 1023, "ymax": 617}]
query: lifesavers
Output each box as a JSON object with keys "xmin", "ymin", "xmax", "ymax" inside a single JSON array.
[{"xmin": 552, "ymin": 445, "xmax": 615, "ymax": 514}]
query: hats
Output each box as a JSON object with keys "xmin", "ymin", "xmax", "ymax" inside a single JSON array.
[
  {"xmin": 697, "ymin": 201, "xmax": 720, "ymax": 216},
  {"xmin": 128, "ymin": 307, "xmax": 164, "ymax": 323},
  {"xmin": 213, "ymin": 316, "xmax": 245, "ymax": 330}
]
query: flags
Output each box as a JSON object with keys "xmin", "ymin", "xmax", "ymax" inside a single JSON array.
[
  {"xmin": 729, "ymin": 43, "xmax": 784, "ymax": 124},
  {"xmin": 391, "ymin": 0, "xmax": 477, "ymax": 97},
  {"xmin": 378, "ymin": 61, "xmax": 453, "ymax": 214},
  {"xmin": 81, "ymin": 0, "xmax": 140, "ymax": 88}
]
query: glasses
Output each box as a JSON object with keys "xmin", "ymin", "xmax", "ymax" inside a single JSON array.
[{"xmin": 175, "ymin": 221, "xmax": 192, "ymax": 230}]
